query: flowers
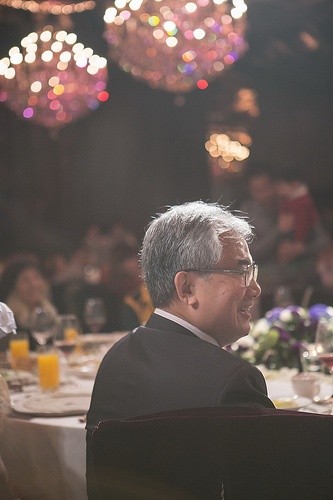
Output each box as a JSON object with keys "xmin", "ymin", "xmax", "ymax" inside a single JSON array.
[{"xmin": 231, "ymin": 303, "xmax": 333, "ymax": 375}]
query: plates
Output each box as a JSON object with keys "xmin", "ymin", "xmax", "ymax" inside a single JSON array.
[
  {"xmin": 269, "ymin": 395, "xmax": 312, "ymax": 411},
  {"xmin": 10, "ymin": 393, "xmax": 92, "ymax": 416}
]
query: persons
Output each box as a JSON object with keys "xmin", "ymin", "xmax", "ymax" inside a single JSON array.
[
  {"xmin": 1, "ymin": 224, "xmax": 154, "ymax": 350},
  {"xmin": 86, "ymin": 202, "xmax": 276, "ymax": 500},
  {"xmin": 240, "ymin": 158, "xmax": 333, "ymax": 320}
]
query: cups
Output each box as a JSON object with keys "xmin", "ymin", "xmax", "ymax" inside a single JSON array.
[
  {"xmin": 36, "ymin": 346, "xmax": 60, "ymax": 392},
  {"xmin": 9, "ymin": 332, "xmax": 29, "ymax": 368},
  {"xmin": 63, "ymin": 320, "xmax": 78, "ymax": 341},
  {"xmin": 291, "ymin": 375, "xmax": 320, "ymax": 398}
]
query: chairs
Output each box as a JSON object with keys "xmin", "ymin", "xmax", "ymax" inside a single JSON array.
[{"xmin": 90, "ymin": 408, "xmax": 333, "ymax": 500}]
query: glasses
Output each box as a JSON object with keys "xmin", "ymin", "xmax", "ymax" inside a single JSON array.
[{"xmin": 184, "ymin": 263, "xmax": 258, "ymax": 286}]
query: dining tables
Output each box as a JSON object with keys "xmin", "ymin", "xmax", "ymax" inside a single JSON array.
[{"xmin": 0, "ymin": 375, "xmax": 333, "ymax": 500}]
